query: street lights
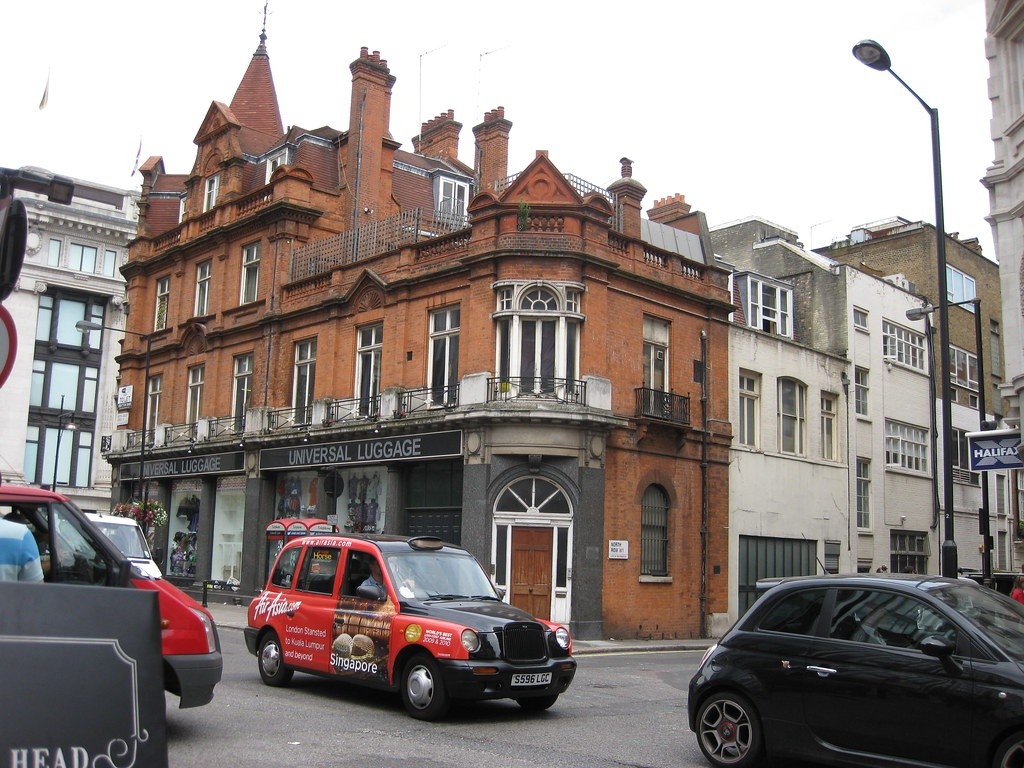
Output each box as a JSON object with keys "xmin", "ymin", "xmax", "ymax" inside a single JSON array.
[
  {"xmin": 76, "ymin": 320, "xmax": 152, "ymax": 502},
  {"xmin": 52, "ymin": 394, "xmax": 79, "ymax": 493},
  {"xmin": 906, "ymin": 298, "xmax": 992, "ymax": 589},
  {"xmin": 850, "ymin": 39, "xmax": 959, "ymax": 579}
]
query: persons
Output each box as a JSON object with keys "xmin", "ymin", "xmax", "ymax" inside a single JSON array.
[
  {"xmin": 4, "ymin": 512, "xmax": 62, "ymax": 573},
  {"xmin": 853, "ymin": 601, "xmax": 888, "ymax": 646},
  {"xmin": 360, "ymin": 556, "xmax": 416, "ymax": 600},
  {"xmin": 900, "ymin": 566, "xmax": 916, "ymax": 574},
  {"xmin": 0, "ymin": 473, "xmax": 45, "ymax": 583},
  {"xmin": 1009, "ymin": 575, "xmax": 1024, "ymax": 605}
]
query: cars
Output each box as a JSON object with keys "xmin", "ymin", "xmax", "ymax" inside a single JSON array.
[
  {"xmin": 244, "ymin": 533, "xmax": 578, "ymax": 721},
  {"xmin": 0, "ymin": 483, "xmax": 224, "ymax": 708},
  {"xmin": 43, "ymin": 512, "xmax": 164, "ymax": 580},
  {"xmin": 686, "ymin": 572, "xmax": 1024, "ymax": 768},
  {"xmin": 961, "ymin": 573, "xmax": 1024, "ymax": 605}
]
